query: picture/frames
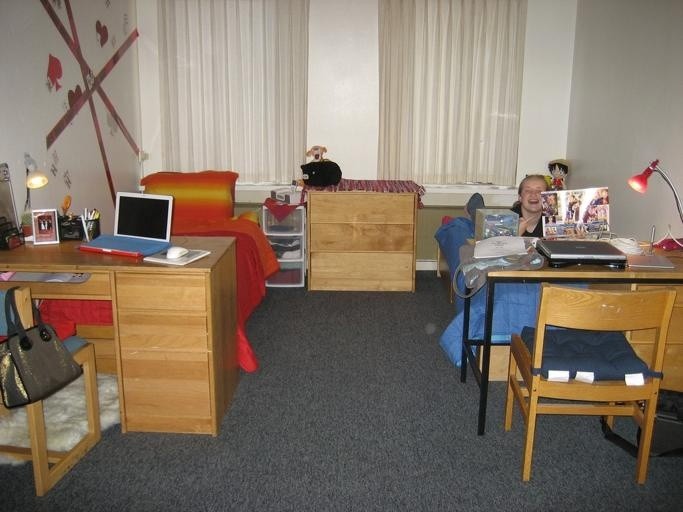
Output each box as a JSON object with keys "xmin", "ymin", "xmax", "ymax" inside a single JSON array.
[{"xmin": 30, "ymin": 208, "xmax": 59, "ymax": 246}]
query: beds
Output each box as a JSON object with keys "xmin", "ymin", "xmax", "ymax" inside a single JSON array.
[
  {"xmin": 435, "ymin": 214, "xmax": 589, "ymax": 384},
  {"xmin": 36, "ymin": 207, "xmax": 280, "ymax": 373}
]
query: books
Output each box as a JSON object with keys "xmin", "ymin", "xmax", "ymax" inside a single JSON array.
[{"xmin": 142, "ymin": 246, "xmax": 211, "ymax": 266}]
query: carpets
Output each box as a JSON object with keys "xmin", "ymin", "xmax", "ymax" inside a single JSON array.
[{"xmin": 0, "ymin": 366, "xmax": 120, "ymax": 469}]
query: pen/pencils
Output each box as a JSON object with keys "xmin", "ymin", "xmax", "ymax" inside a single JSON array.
[
  {"xmin": 80, "ymin": 208, "xmax": 100, "ymax": 242},
  {"xmin": 649, "ymin": 225, "xmax": 656, "ymax": 255}
]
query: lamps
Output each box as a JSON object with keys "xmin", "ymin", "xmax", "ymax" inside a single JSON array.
[
  {"xmin": 626, "ymin": 158, "xmax": 683, "ymax": 226},
  {"xmin": 19, "ymin": 152, "xmax": 50, "ymax": 230}
]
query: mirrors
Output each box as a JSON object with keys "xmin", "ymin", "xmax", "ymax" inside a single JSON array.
[{"xmin": 0, "ymin": 163, "xmax": 23, "ymax": 249}]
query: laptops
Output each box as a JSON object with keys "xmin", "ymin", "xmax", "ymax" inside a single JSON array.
[
  {"xmin": 536, "ymin": 240, "xmax": 628, "ymax": 265},
  {"xmin": 80, "ymin": 191, "xmax": 173, "ymax": 258}
]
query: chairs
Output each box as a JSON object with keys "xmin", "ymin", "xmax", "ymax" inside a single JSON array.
[
  {"xmin": 0, "ymin": 286, "xmax": 102, "ymax": 497},
  {"xmin": 501, "ymin": 280, "xmax": 677, "ymax": 487}
]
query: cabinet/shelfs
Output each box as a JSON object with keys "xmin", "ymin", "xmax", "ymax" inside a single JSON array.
[
  {"xmin": 304, "ymin": 178, "xmax": 419, "ymax": 294},
  {"xmin": 259, "ymin": 204, "xmax": 305, "ymax": 290}
]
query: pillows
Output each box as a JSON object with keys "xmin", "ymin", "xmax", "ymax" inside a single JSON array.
[{"xmin": 140, "ymin": 168, "xmax": 239, "ymax": 221}]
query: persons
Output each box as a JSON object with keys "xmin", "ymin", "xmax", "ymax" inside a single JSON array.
[
  {"xmin": 509, "ymin": 175, "xmax": 549, "ymax": 238},
  {"xmin": 548, "ymin": 159, "xmax": 569, "ymax": 191}
]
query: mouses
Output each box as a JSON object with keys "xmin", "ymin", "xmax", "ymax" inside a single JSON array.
[{"xmin": 166, "ymin": 245, "xmax": 188, "ymax": 258}]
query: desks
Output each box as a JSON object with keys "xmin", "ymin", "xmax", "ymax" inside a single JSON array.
[
  {"xmin": 0, "ymin": 231, "xmax": 238, "ymax": 438},
  {"xmin": 457, "ymin": 238, "xmax": 683, "ymax": 439}
]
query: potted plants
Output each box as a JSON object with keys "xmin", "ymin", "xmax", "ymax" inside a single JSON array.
[{"xmin": 19, "ymin": 210, "xmax": 34, "ymax": 238}]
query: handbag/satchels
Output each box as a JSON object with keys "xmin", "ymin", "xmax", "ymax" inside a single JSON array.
[
  {"xmin": 452, "ymin": 238, "xmax": 545, "ymax": 300},
  {"xmin": 1, "ymin": 285, "xmax": 86, "ymax": 410}
]
